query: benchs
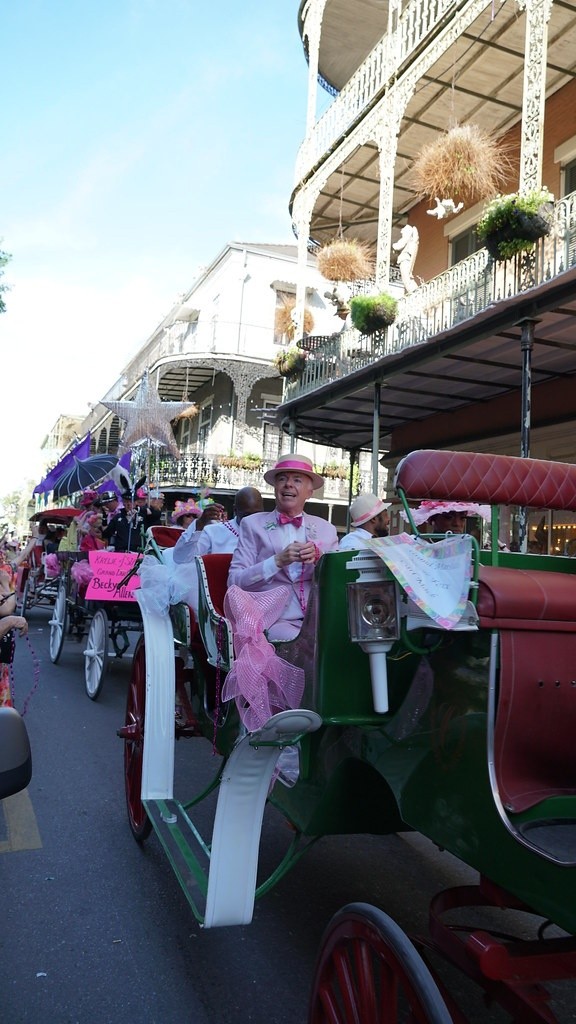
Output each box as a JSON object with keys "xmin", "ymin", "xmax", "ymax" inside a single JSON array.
[
  {"xmin": 146, "ymin": 525, "xmax": 206, "ymax": 647},
  {"xmin": 41, "ymin": 539, "xmax": 73, "ymax": 581},
  {"xmin": 196, "ymin": 554, "xmax": 235, "ymax": 672},
  {"xmin": 392, "ymin": 450, "xmax": 576, "ymax": 816}
]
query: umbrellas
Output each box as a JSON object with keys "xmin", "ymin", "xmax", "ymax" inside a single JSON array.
[{"xmin": 53, "ymin": 454, "xmax": 119, "ymax": 498}]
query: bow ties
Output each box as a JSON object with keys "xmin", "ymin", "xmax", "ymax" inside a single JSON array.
[
  {"xmin": 279, "ymin": 513, "xmax": 302, "ymax": 528},
  {"xmin": 111, "ymin": 511, "xmax": 117, "ymax": 517}
]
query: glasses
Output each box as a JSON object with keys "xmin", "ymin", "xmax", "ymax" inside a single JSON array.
[{"xmin": 185, "ymin": 513, "xmax": 197, "ymax": 519}]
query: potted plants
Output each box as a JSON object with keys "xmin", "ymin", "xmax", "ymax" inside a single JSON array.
[{"xmin": 347, "ymin": 290, "xmax": 397, "ymax": 336}]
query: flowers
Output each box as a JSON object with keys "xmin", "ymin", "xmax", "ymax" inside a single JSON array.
[
  {"xmin": 473, "ymin": 184, "xmax": 555, "ymax": 240},
  {"xmin": 87, "ymin": 513, "xmax": 102, "ymax": 526},
  {"xmin": 272, "ymin": 347, "xmax": 298, "ymax": 373}
]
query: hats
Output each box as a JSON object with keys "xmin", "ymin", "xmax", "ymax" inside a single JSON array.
[
  {"xmin": 399, "ymin": 500, "xmax": 491, "ymax": 527},
  {"xmin": 80, "ymin": 490, "xmax": 99, "ymax": 506},
  {"xmin": 6, "ymin": 542, "xmax": 17, "ymax": 546},
  {"xmin": 150, "ymin": 490, "xmax": 165, "ymax": 499},
  {"xmin": 195, "ymin": 499, "xmax": 215, "ymax": 511},
  {"xmin": 170, "ymin": 498, "xmax": 202, "ymax": 524},
  {"xmin": 100, "ymin": 491, "xmax": 116, "ymax": 503},
  {"xmin": 119, "ymin": 473, "xmax": 147, "ymax": 500},
  {"xmin": 137, "ymin": 488, "xmax": 149, "ymax": 499},
  {"xmin": 349, "ymin": 492, "xmax": 392, "ymax": 527},
  {"xmin": 264, "ymin": 454, "xmax": 324, "ymax": 490}
]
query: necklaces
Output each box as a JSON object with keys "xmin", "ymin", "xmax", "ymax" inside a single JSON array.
[
  {"xmin": 429, "ymin": 538, "xmax": 434, "ymax": 543},
  {"xmin": 217, "ymin": 508, "xmax": 239, "ymax": 537},
  {"xmin": 300, "ymin": 541, "xmax": 320, "ymax": 615}
]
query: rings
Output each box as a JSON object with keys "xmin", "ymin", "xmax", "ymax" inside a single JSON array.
[{"xmin": 297, "ymin": 552, "xmax": 299, "ymax": 556}]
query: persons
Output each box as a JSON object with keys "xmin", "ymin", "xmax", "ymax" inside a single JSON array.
[
  {"xmin": 399, "ymin": 501, "xmax": 491, "ymax": 543},
  {"xmin": 392, "ymin": 224, "xmax": 418, "ymax": 292},
  {"xmin": 77, "ymin": 490, "xmax": 161, "ymax": 553},
  {"xmin": 172, "ymin": 498, "xmax": 201, "ymax": 529},
  {"xmin": 173, "ymin": 487, "xmax": 264, "ymax": 565},
  {"xmin": 0, "ymin": 521, "xmax": 37, "ymax": 639},
  {"xmin": 227, "ymin": 454, "xmax": 338, "ymax": 641},
  {"xmin": 338, "ymin": 493, "xmax": 392, "ymax": 551}
]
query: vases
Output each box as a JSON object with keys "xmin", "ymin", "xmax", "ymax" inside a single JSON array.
[
  {"xmin": 485, "ymin": 204, "xmax": 554, "ymax": 261},
  {"xmin": 280, "ymin": 354, "xmax": 307, "ymax": 376}
]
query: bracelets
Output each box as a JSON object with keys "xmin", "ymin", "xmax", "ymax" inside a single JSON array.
[{"xmin": 32, "ymin": 537, "xmax": 38, "ymax": 539}]
